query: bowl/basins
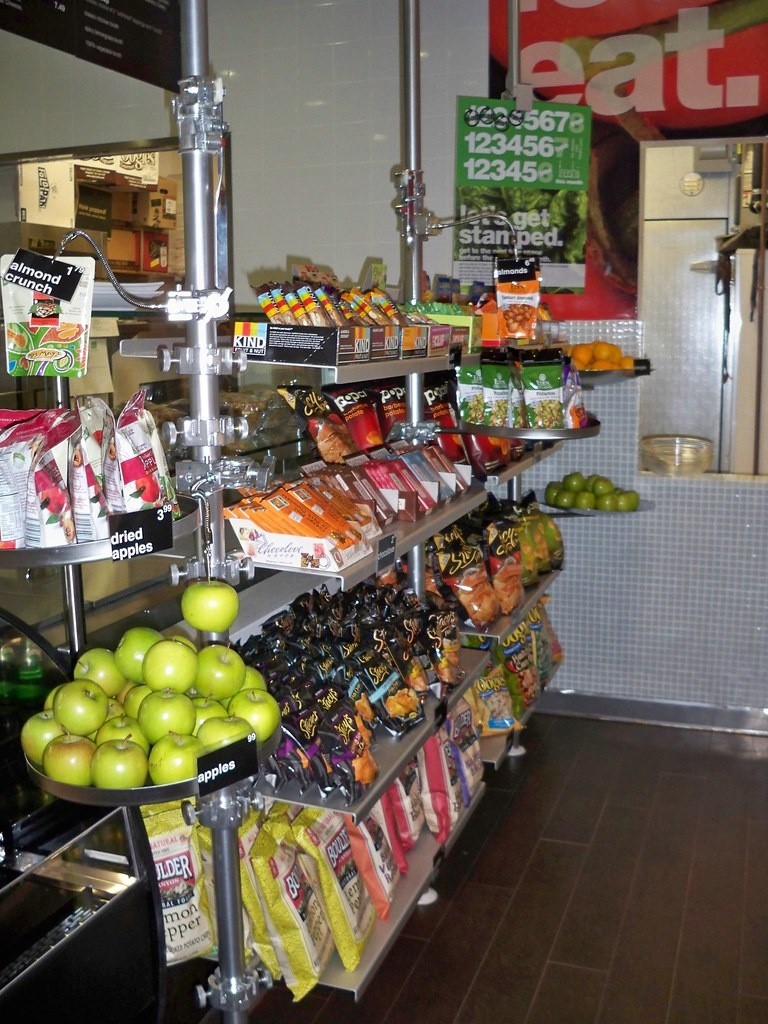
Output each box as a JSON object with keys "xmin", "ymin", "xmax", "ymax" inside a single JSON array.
[{"xmin": 640, "ymin": 437, "xmax": 713, "ymax": 477}]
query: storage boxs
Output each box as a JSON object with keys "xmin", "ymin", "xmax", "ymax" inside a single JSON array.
[
  {"xmin": 132, "ymin": 192, "xmax": 177, "ymax": 230},
  {"xmin": 76, "ymin": 184, "xmax": 112, "ymax": 240},
  {"xmin": 0, "ymin": 159, "xmax": 75, "ymax": 228},
  {"xmin": 135, "ymin": 231, "xmax": 168, "ymax": 273},
  {"xmin": 74, "ymin": 155, "xmax": 116, "ymax": 185},
  {"xmin": 158, "ymin": 176, "xmax": 178, "ymax": 194},
  {"xmin": 109, "ymin": 151, "xmax": 159, "ymax": 192},
  {"xmin": 0, "ymin": 224, "xmax": 108, "ymax": 282},
  {"xmin": 108, "ymin": 230, "xmax": 135, "ymax": 273},
  {"xmin": 111, "ymin": 191, "xmax": 132, "ymax": 229}
]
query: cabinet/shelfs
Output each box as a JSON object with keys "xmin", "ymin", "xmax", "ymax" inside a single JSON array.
[{"xmin": 0, "ymin": 283, "xmax": 654, "ymax": 1024}]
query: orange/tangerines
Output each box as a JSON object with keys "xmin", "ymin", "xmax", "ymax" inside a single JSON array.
[{"xmin": 569, "ymin": 340, "xmax": 635, "ymax": 371}]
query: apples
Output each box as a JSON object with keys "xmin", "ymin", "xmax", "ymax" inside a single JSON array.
[
  {"xmin": 545, "ymin": 472, "xmax": 639, "ymax": 511},
  {"xmin": 180, "ymin": 581, "xmax": 240, "ymax": 633},
  {"xmin": 21, "ymin": 627, "xmax": 280, "ymax": 788}
]
